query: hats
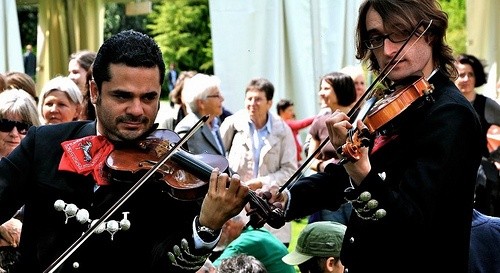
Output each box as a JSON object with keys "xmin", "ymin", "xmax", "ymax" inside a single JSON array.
[{"xmin": 281, "ymin": 221, "xmax": 347, "ymax": 266}]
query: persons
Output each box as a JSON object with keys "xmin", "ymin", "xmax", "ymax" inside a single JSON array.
[
  {"xmin": 166, "ymin": 61, "xmax": 180, "ymax": 109},
  {"xmin": 197, "ymin": 53, "xmax": 500, "ymax": 273},
  {"xmin": 245, "ymin": 0, "xmax": 484, "ymax": 273},
  {"xmin": 0, "ymin": 29, "xmax": 262, "ymax": 273},
  {"xmin": 23, "ymin": 45, "xmax": 36, "ymax": 83}
]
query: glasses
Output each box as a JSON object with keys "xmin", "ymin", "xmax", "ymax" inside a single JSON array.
[
  {"xmin": 0, "ymin": 118, "xmax": 33, "ymax": 135},
  {"xmin": 207, "ymin": 94, "xmax": 221, "ymax": 99},
  {"xmin": 362, "ymin": 23, "xmax": 423, "ymax": 50}
]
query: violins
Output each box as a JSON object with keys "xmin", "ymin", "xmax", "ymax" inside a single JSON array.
[
  {"xmin": 105, "ymin": 129, "xmax": 285, "ymax": 230},
  {"xmin": 336, "ymin": 74, "xmax": 433, "ymax": 165}
]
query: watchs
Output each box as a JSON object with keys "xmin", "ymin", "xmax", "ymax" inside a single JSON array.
[{"xmin": 195, "ymin": 216, "xmax": 222, "ymax": 242}]
